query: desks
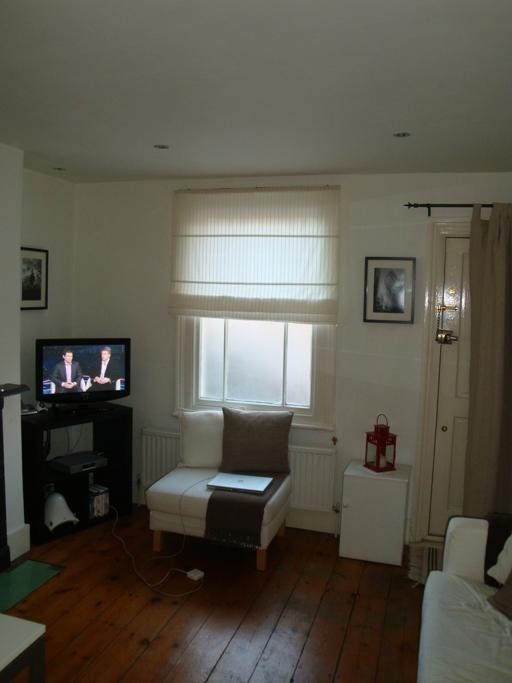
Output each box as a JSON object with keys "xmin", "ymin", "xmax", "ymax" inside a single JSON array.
[{"xmin": 0, "ymin": 614, "xmax": 47, "ymax": 683}]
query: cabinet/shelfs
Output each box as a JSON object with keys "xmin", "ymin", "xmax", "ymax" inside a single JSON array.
[
  {"xmin": 339, "ymin": 460, "xmax": 412, "ymax": 566},
  {"xmin": 21, "ymin": 402, "xmax": 133, "ymax": 545}
]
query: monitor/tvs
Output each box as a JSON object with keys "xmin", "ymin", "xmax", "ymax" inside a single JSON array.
[{"xmin": 35, "ymin": 337, "xmax": 131, "ymax": 417}]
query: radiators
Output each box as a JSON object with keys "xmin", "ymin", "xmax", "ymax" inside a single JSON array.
[{"xmin": 139, "ymin": 427, "xmax": 340, "ymax": 514}]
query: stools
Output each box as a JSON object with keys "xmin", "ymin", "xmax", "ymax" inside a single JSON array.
[{"xmin": 145, "ymin": 467, "xmax": 292, "ymax": 572}]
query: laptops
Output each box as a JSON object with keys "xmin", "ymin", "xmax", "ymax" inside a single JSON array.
[{"xmin": 206, "ymin": 471, "xmax": 274, "ymax": 495}]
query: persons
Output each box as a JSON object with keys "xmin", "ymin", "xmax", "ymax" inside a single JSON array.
[
  {"xmin": 85, "ymin": 347, "xmax": 120, "ymax": 392},
  {"xmin": 49, "ymin": 349, "xmax": 82, "ymax": 393},
  {"xmin": 376, "ymin": 269, "xmax": 403, "ymax": 313}
]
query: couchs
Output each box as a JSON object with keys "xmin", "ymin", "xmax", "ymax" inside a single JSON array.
[{"xmin": 413, "ymin": 514, "xmax": 511, "ymax": 682}]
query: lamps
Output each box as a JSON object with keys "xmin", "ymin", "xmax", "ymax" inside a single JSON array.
[{"xmin": 362, "ymin": 412, "xmax": 399, "ymax": 475}]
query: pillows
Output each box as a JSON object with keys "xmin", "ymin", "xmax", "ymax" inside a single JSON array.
[
  {"xmin": 218, "ymin": 406, "xmax": 294, "ymax": 474},
  {"xmin": 176, "ymin": 410, "xmax": 224, "ymax": 469}
]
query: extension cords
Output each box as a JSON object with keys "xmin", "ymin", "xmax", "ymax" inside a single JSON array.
[{"xmin": 187, "ymin": 568, "xmax": 205, "ymax": 580}]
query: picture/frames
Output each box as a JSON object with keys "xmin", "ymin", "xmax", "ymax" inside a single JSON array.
[
  {"xmin": 20, "ymin": 247, "xmax": 48, "ymax": 310},
  {"xmin": 363, "ymin": 254, "xmax": 416, "ymax": 324}
]
